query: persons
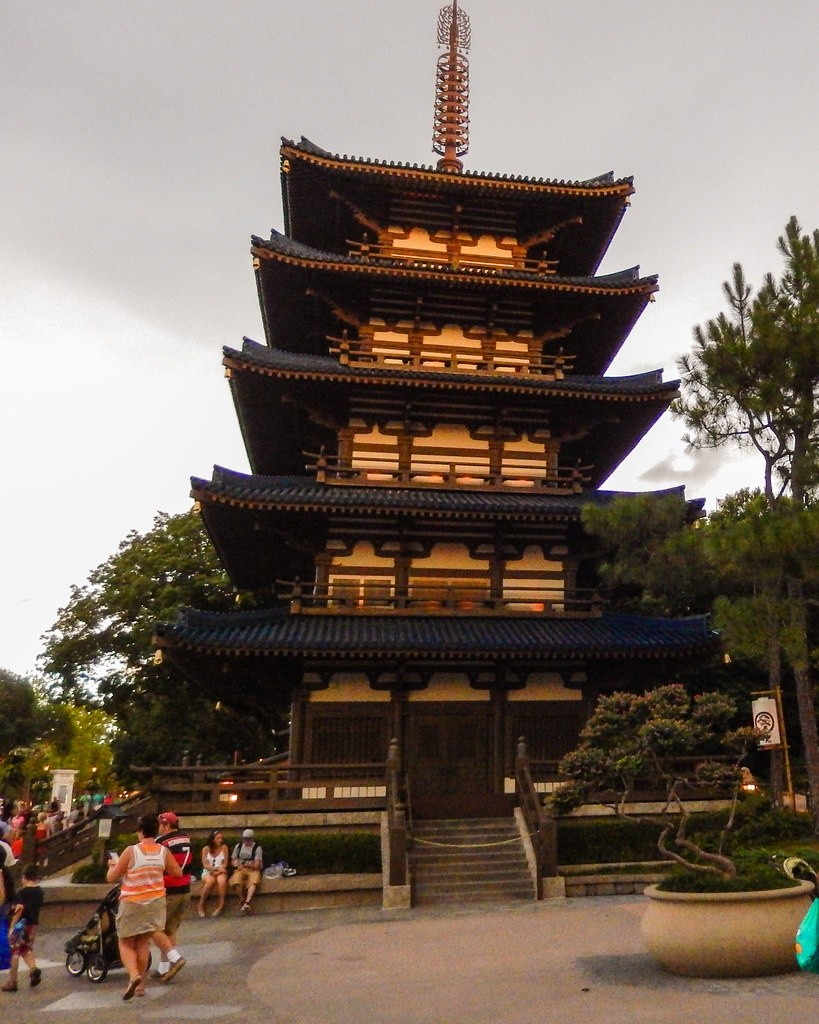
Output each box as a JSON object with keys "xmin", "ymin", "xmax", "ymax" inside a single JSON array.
[
  {"xmin": 105, "ymin": 815, "xmax": 182, "ymax": 999},
  {"xmin": 0, "ymin": 864, "xmax": 43, "ymax": 992},
  {"xmin": 197, "ymin": 830, "xmax": 229, "ymax": 917},
  {"xmin": 153, "ymin": 812, "xmax": 191, "ymax": 981},
  {"xmin": 0, "ymin": 789, "xmax": 113, "ymax": 864},
  {"xmin": 228, "ymin": 830, "xmax": 262, "ymax": 912}
]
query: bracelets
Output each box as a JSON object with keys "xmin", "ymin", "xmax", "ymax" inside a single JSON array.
[{"xmin": 110, "ymin": 863, "xmax": 116, "ymax": 866}]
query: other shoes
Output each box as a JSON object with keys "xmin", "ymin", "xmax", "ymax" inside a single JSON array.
[
  {"xmin": 30, "ymin": 968, "xmax": 41, "ymax": 987},
  {"xmin": 136, "ymin": 984, "xmax": 145, "ymax": 996},
  {"xmin": 123, "ymin": 974, "xmax": 142, "ymax": 1000},
  {"xmin": 197, "ymin": 904, "xmax": 205, "ymax": 917},
  {"xmin": 0, "ymin": 982, "xmax": 17, "ymax": 991},
  {"xmin": 239, "ymin": 897, "xmax": 251, "ymax": 914},
  {"xmin": 212, "ymin": 912, "xmax": 222, "ymax": 916}
]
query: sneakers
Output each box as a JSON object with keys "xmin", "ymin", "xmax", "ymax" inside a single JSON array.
[
  {"xmin": 147, "ymin": 970, "xmax": 163, "ymax": 980},
  {"xmin": 161, "ymin": 956, "xmax": 186, "ymax": 981}
]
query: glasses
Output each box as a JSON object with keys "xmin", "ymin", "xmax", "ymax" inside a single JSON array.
[{"xmin": 212, "ymin": 857, "xmax": 216, "ymax": 866}]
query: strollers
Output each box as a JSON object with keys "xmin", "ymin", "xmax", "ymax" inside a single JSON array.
[{"xmin": 64, "ymin": 885, "xmax": 152, "ymax": 985}]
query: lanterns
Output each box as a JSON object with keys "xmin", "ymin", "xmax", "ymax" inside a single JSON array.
[{"xmin": 752, "ymin": 698, "xmax": 780, "ymax": 746}]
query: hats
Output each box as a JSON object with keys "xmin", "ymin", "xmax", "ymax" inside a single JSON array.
[
  {"xmin": 243, "ymin": 829, "xmax": 254, "ymax": 837},
  {"xmin": 157, "ymin": 812, "xmax": 179, "ymax": 824}
]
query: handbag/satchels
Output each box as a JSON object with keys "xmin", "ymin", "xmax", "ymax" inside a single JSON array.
[{"xmin": 795, "ymin": 898, "xmax": 819, "ymax": 974}]
